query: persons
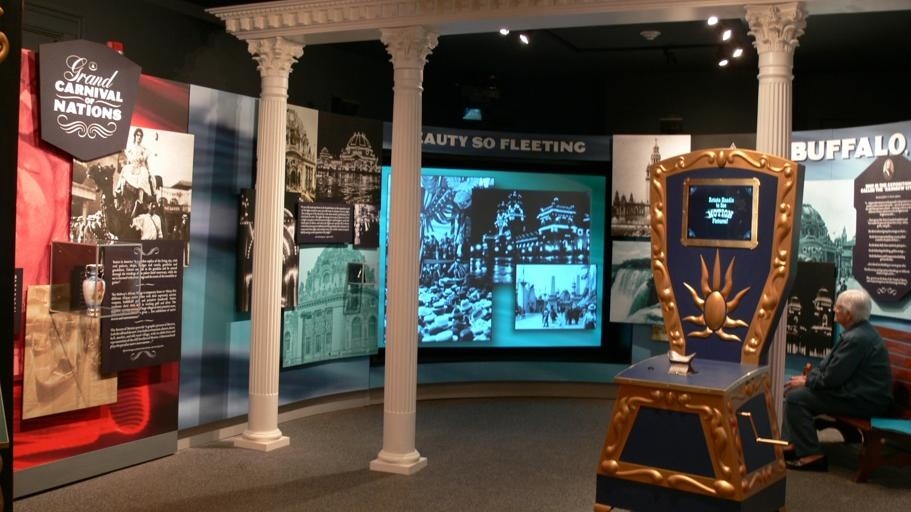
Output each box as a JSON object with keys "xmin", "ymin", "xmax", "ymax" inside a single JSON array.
[
  {"xmin": 116, "ymin": 126, "xmax": 166, "ymax": 243},
  {"xmin": 780, "ymin": 287, "xmax": 896, "ymax": 469},
  {"xmin": 408, "ymin": 190, "xmax": 596, "ymax": 347}
]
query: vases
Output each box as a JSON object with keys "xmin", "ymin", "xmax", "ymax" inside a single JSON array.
[{"xmin": 82, "ymin": 264, "xmax": 106, "ymax": 312}]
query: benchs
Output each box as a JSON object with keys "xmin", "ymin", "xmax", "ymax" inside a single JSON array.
[{"xmin": 827, "ymin": 326, "xmax": 910, "ymax": 484}]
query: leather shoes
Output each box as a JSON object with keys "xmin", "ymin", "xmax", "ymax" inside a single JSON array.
[{"xmin": 782, "ymin": 444, "xmax": 830, "ymax": 472}]
